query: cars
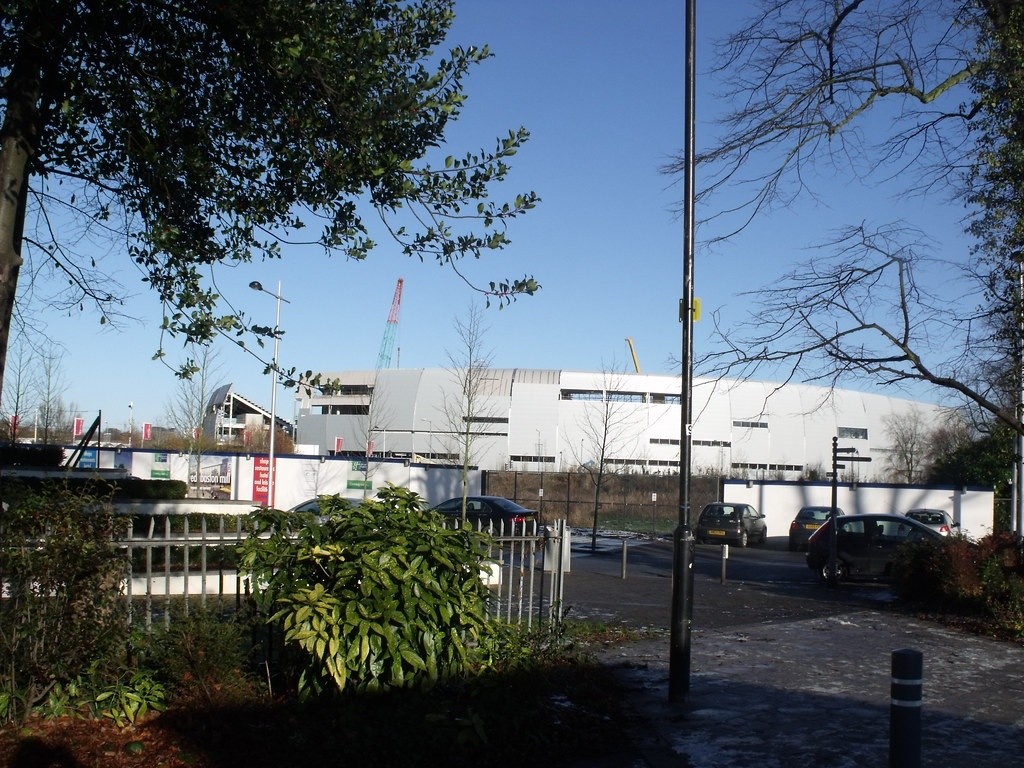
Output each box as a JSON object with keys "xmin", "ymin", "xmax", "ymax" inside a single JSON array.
[
  {"xmin": 695, "ymin": 502, "xmax": 767, "ymax": 547},
  {"xmin": 284, "ymin": 498, "xmax": 383, "ymax": 532},
  {"xmin": 401, "ymin": 496, "xmax": 539, "ymax": 545},
  {"xmin": 897, "ymin": 509, "xmax": 961, "ymax": 539},
  {"xmin": 790, "ymin": 505, "xmax": 852, "ymax": 551},
  {"xmin": 805, "ymin": 514, "xmax": 949, "ymax": 586}
]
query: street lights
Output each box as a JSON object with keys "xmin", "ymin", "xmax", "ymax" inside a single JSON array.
[{"xmin": 249, "ymin": 281, "xmax": 282, "ymax": 512}]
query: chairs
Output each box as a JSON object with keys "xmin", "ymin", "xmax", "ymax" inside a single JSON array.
[
  {"xmin": 873, "ymin": 525, "xmax": 888, "ymax": 539},
  {"xmin": 806, "ymin": 511, "xmax": 818, "ymax": 519},
  {"xmin": 921, "ymin": 515, "xmax": 941, "ymax": 521},
  {"xmin": 467, "ymin": 504, "xmax": 474, "ymax": 511}
]
